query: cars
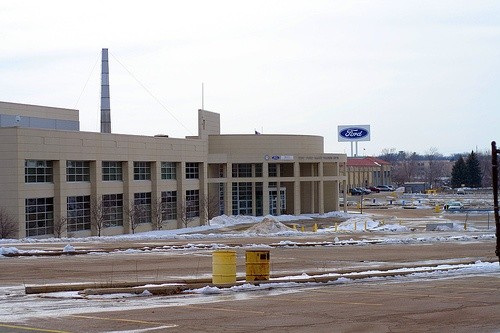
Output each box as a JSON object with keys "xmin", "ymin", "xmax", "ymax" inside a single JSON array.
[
  {"xmin": 367, "ymin": 186, "xmax": 381, "ymax": 192},
  {"xmin": 457, "ymin": 188, "xmax": 465, "ymax": 195},
  {"xmin": 442, "ymin": 201, "xmax": 464, "ymax": 210},
  {"xmin": 342, "ymin": 188, "xmax": 362, "ymax": 195},
  {"xmin": 402, "ymin": 201, "xmax": 417, "ymax": 209},
  {"xmin": 356, "ymin": 187, "xmax": 372, "ymax": 194}
]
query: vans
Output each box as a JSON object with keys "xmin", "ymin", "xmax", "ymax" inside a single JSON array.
[{"xmin": 376, "ymin": 184, "xmax": 395, "ymax": 191}]
